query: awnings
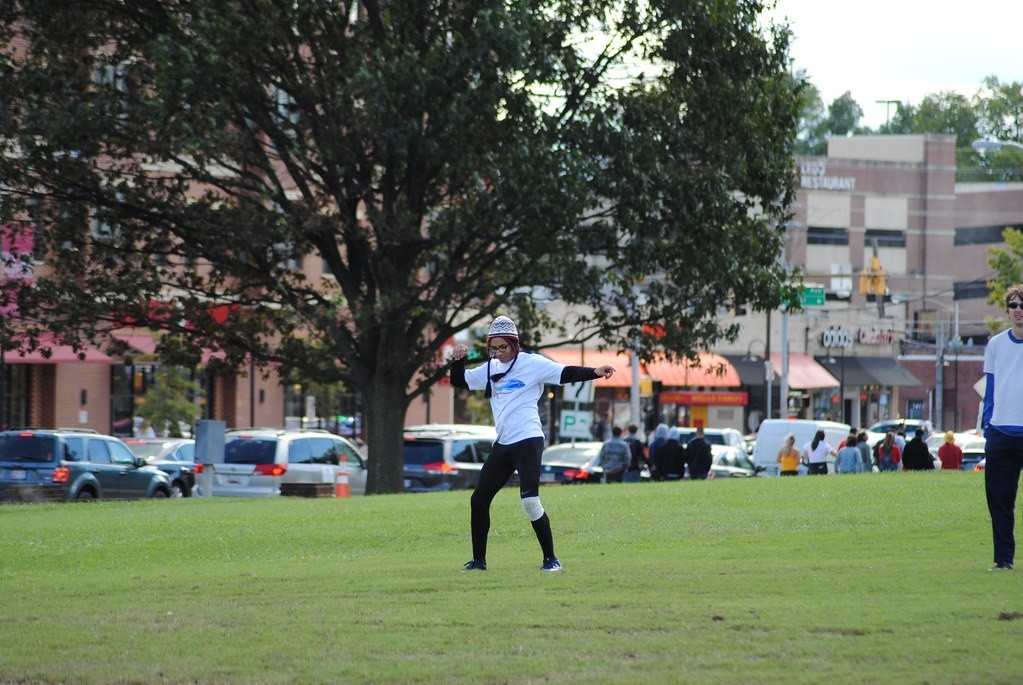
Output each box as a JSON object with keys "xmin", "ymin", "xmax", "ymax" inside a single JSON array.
[
  {"xmin": 535, "ymin": 347, "xmax": 925, "ymax": 391},
  {"xmin": 2, "ymin": 328, "xmax": 258, "ymax": 365}
]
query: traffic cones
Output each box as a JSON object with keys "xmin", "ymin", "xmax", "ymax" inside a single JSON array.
[{"xmin": 335, "ymin": 453, "xmax": 350, "ymax": 497}]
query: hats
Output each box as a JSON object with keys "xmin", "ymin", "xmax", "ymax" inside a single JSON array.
[
  {"xmin": 944, "ymin": 430, "xmax": 955, "ymax": 443},
  {"xmin": 485, "ymin": 316, "xmax": 519, "ymax": 398}
]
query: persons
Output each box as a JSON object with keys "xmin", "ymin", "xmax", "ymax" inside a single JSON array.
[
  {"xmin": 450, "ymin": 315, "xmax": 617, "ymax": 573},
  {"xmin": 598, "ymin": 421, "xmax": 712, "ymax": 483},
  {"xmin": 981, "ymin": 289, "xmax": 1023, "ymax": 571},
  {"xmin": 777, "ymin": 426, "xmax": 964, "ymax": 476}
]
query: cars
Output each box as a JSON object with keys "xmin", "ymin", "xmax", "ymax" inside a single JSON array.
[
  {"xmin": 538, "ymin": 441, "xmax": 652, "ymax": 485},
  {"xmin": 868, "ymin": 419, "xmax": 987, "ymax": 471},
  {"xmin": 118, "ymin": 436, "xmax": 196, "ymax": 499},
  {"xmin": 669, "ymin": 425, "xmax": 758, "ymax": 480}
]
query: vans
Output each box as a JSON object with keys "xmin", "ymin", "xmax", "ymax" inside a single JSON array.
[{"xmin": 752, "ymin": 417, "xmax": 886, "ymax": 475}]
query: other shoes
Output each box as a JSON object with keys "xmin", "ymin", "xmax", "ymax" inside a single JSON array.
[
  {"xmin": 988, "ymin": 562, "xmax": 1014, "ymax": 571},
  {"xmin": 540, "ymin": 559, "xmax": 562, "ymax": 572},
  {"xmin": 463, "ymin": 559, "xmax": 487, "ymax": 572}
]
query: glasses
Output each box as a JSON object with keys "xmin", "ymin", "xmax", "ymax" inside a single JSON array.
[
  {"xmin": 489, "ymin": 344, "xmax": 510, "ymax": 352},
  {"xmin": 1008, "ymin": 302, "xmax": 1023, "ymax": 309}
]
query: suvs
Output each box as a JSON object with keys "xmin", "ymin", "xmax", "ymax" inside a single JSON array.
[
  {"xmin": 0, "ymin": 427, "xmax": 170, "ymax": 502},
  {"xmin": 400, "ymin": 423, "xmax": 524, "ymax": 492},
  {"xmin": 191, "ymin": 426, "xmax": 367, "ymax": 497}
]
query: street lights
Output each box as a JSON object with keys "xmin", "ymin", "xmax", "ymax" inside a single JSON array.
[{"xmin": 890, "ymin": 294, "xmax": 961, "ymax": 338}]
